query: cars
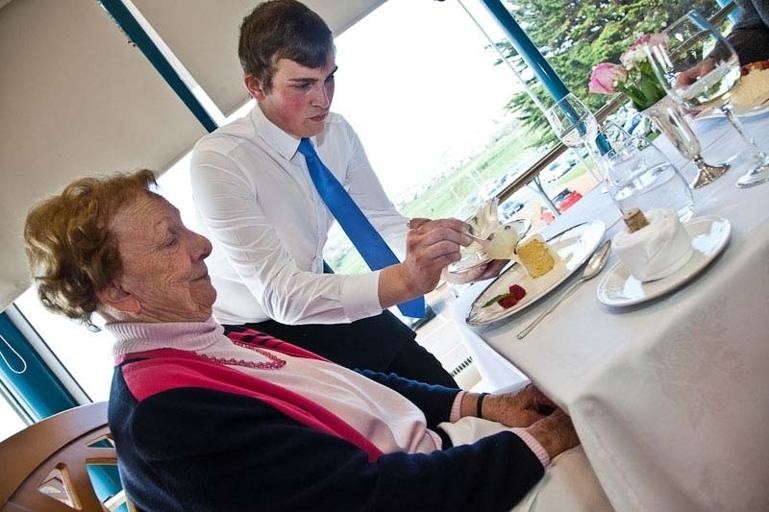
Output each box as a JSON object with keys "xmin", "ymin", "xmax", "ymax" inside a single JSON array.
[
  {"xmin": 447, "ymin": 144, "xmax": 587, "ymax": 226},
  {"xmin": 598, "ymin": 73, "xmax": 674, "ymax": 144}
]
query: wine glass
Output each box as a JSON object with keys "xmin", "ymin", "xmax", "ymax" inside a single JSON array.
[{"xmin": 650, "ymin": 12, "xmax": 768, "ymax": 192}]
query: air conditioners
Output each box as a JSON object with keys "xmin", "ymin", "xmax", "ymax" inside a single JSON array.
[{"xmin": 443, "ymin": 345, "xmax": 483, "ymax": 392}]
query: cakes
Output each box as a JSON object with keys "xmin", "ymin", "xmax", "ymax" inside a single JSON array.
[{"xmin": 611, "ymin": 204, "xmax": 695, "ymax": 283}]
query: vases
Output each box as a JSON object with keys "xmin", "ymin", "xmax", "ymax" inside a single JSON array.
[{"xmin": 637, "ymin": 94, "xmax": 732, "ymax": 190}]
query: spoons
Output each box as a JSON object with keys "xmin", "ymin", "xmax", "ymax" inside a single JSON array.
[
  {"xmin": 407, "ymin": 216, "xmax": 521, "ymax": 255},
  {"xmin": 514, "ymin": 240, "xmax": 611, "ymax": 342}
]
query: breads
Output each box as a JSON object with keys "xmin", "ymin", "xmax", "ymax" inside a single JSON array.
[{"xmin": 514, "ymin": 234, "xmax": 556, "ymax": 279}]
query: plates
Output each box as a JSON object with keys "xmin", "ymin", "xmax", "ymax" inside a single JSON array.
[{"xmin": 442, "ymin": 76, "xmax": 769, "ymax": 324}]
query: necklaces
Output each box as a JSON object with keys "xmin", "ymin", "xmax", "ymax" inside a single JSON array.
[{"xmin": 188, "ymin": 339, "xmax": 286, "ymax": 370}]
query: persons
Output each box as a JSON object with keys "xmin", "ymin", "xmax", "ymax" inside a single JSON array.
[
  {"xmin": 22, "ymin": 166, "xmax": 617, "ymax": 512},
  {"xmin": 183, "ymin": 0, "xmax": 517, "ymax": 389},
  {"xmin": 671, "ymin": 0, "xmax": 768, "ymax": 93}
]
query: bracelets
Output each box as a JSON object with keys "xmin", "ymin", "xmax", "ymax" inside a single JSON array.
[{"xmin": 475, "ymin": 391, "xmax": 494, "ymax": 418}]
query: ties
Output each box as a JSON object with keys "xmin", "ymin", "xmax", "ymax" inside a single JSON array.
[{"xmin": 296, "ymin": 137, "xmax": 426, "ymax": 318}]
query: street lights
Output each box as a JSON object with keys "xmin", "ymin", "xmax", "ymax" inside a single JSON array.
[{"xmin": 435, "ymin": 0, "xmax": 565, "ymax": 134}]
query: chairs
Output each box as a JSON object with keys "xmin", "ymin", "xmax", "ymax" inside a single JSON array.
[{"xmin": 0, "ymin": 398, "xmax": 142, "ymax": 512}]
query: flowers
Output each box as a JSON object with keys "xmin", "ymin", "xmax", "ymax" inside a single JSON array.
[{"xmin": 583, "ymin": 33, "xmax": 677, "ymax": 109}]
